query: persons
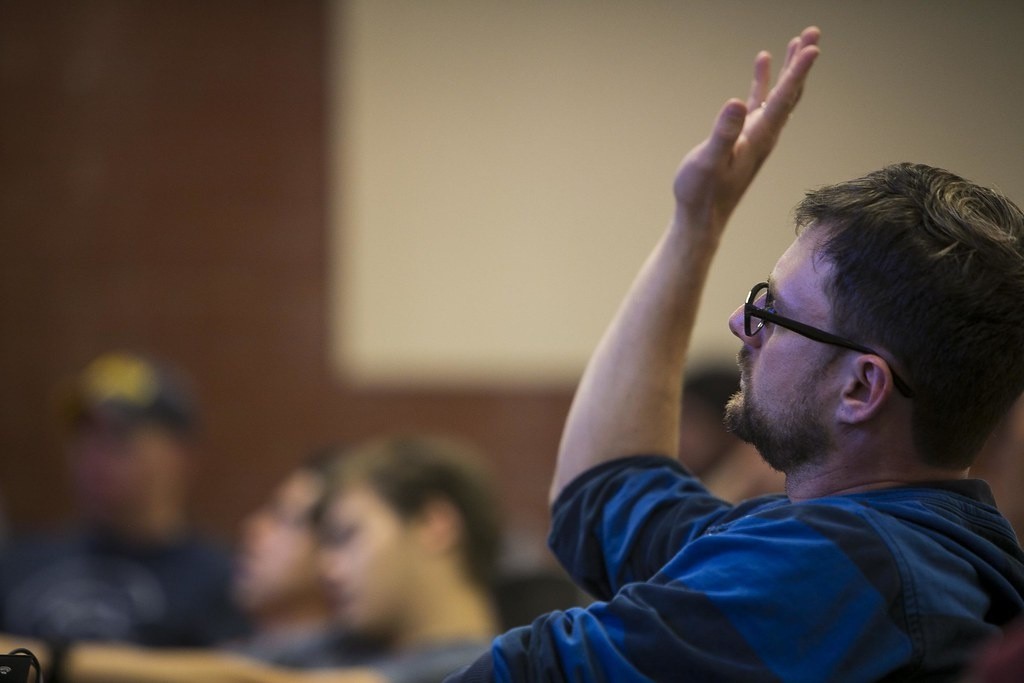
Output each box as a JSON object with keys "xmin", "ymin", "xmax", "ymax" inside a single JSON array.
[
  {"xmin": 3, "ymin": 349, "xmax": 260, "ymax": 656},
  {"xmin": 449, "ymin": 18, "xmax": 1024, "ymax": 683},
  {"xmin": 212, "ymin": 446, "xmax": 368, "ymax": 664},
  {"xmin": 0, "ymin": 433, "xmax": 504, "ymax": 683},
  {"xmin": 670, "ymin": 360, "xmax": 780, "ymax": 505}
]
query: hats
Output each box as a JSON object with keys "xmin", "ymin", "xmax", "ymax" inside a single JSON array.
[{"xmin": 67, "ymin": 348, "xmax": 200, "ymax": 430}]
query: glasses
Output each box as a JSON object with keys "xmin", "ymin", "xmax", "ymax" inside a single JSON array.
[{"xmin": 743, "ymin": 282, "xmax": 916, "ymax": 400}]
